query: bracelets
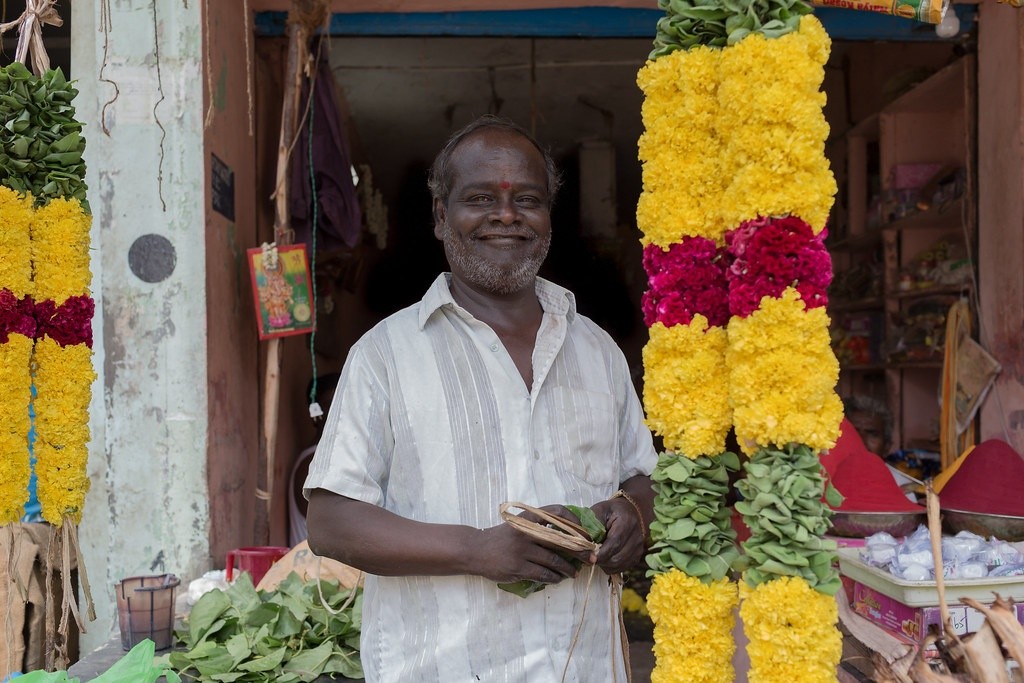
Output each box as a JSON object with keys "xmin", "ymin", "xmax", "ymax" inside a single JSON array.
[{"xmin": 610, "ymin": 489, "xmax": 647, "ymax": 537}]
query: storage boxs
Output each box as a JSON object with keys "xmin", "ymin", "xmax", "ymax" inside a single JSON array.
[{"xmin": 835, "ymin": 546, "xmax": 1024, "ymax": 653}]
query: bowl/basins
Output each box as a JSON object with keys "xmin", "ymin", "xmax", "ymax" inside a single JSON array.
[
  {"xmin": 818, "ymin": 507, "xmax": 929, "ymax": 539},
  {"xmin": 939, "ymin": 507, "xmax": 1024, "ymax": 546}
]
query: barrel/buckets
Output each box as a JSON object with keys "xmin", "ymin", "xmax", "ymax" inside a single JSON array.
[{"xmin": 114, "ymin": 573, "xmax": 181, "ymax": 651}]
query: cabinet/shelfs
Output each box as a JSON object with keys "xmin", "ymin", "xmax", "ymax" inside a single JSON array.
[{"xmin": 826, "ymin": 56, "xmax": 977, "ymax": 472}]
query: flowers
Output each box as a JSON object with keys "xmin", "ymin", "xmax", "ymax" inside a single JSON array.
[
  {"xmin": 638, "ymin": 0, "xmax": 845, "ymax": 683},
  {"xmin": 0, "ymin": 58, "xmax": 94, "ymax": 527}
]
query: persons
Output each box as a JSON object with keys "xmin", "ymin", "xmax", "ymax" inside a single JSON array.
[{"xmin": 302, "ymin": 115, "xmax": 660, "ymax": 683}]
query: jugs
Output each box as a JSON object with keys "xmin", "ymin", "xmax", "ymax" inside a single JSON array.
[{"xmin": 226, "ymin": 546, "xmax": 290, "ymax": 589}]
